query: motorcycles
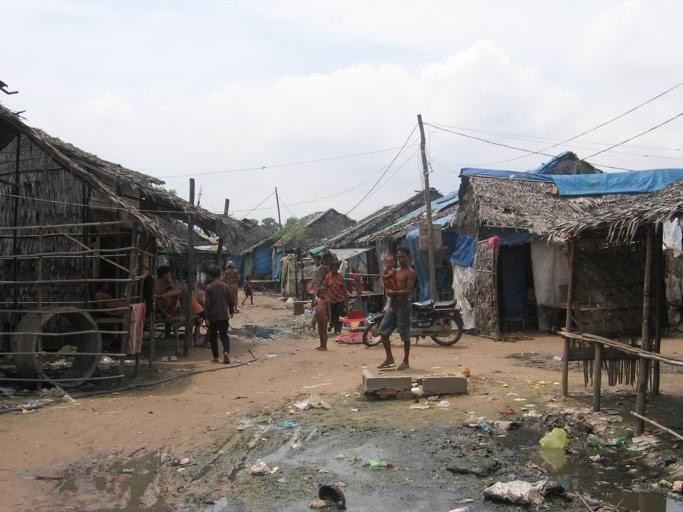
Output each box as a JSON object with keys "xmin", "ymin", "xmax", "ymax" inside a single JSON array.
[{"xmin": 363, "ymin": 297, "xmax": 464, "ymax": 347}]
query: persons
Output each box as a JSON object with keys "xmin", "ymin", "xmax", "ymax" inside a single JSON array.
[
  {"xmin": 377, "ymin": 247, "xmax": 416, "ymax": 370},
  {"xmin": 152, "ymin": 260, "xmax": 255, "ymax": 363},
  {"xmin": 383, "ymin": 254, "xmax": 396, "ymax": 314},
  {"xmin": 312, "ymin": 286, "xmax": 332, "ymax": 351},
  {"xmin": 325, "ymin": 261, "xmax": 348, "ymax": 335}
]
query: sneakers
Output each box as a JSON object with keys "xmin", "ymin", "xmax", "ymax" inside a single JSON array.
[
  {"xmin": 376, "ymin": 358, "xmax": 409, "ymax": 371},
  {"xmin": 210, "ymin": 352, "xmax": 230, "ymax": 363}
]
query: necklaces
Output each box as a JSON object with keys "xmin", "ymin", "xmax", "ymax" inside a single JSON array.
[{"xmin": 92, "ymin": 276, "xmax": 129, "ymax": 318}]
